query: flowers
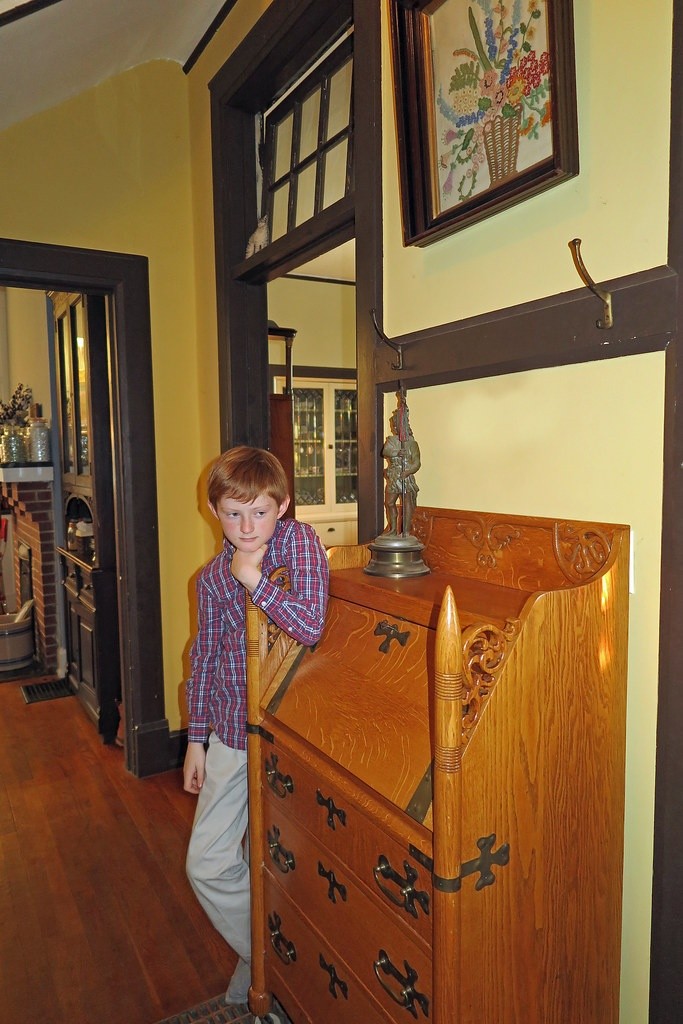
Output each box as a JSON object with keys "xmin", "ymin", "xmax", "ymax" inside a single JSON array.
[{"xmin": 0, "ymin": 381, "xmax": 33, "ymax": 421}]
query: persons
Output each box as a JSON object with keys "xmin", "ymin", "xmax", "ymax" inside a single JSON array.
[
  {"xmin": 183, "ymin": 446, "xmax": 328, "ymax": 1005},
  {"xmin": 382, "ymin": 404, "xmax": 420, "ymax": 537}
]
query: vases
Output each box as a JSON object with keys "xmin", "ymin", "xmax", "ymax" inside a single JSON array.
[{"xmin": 2, "ymin": 418, "xmax": 26, "ymax": 430}]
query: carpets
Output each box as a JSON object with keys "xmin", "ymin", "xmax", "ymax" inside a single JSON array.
[{"xmin": 153, "ymin": 991, "xmax": 291, "ymax": 1024}]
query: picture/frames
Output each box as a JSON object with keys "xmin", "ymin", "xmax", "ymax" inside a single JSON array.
[{"xmin": 386, "ymin": 0, "xmax": 580, "ymax": 248}]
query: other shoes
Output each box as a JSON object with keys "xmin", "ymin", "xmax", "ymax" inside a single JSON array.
[{"xmin": 225, "ymin": 958, "xmax": 251, "ymax": 1004}]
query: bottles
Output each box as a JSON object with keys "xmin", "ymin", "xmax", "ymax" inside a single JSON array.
[{"xmin": 68, "ymin": 522, "xmax": 78, "ymax": 550}]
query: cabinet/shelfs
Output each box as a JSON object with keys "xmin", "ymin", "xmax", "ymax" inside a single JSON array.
[
  {"xmin": 269, "ymin": 364, "xmax": 359, "ymax": 547},
  {"xmin": 44, "ymin": 286, "xmax": 121, "ymax": 745},
  {"xmin": 246, "ymin": 506, "xmax": 633, "ymax": 1024}
]
